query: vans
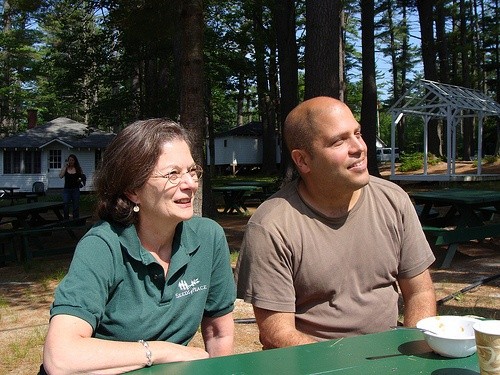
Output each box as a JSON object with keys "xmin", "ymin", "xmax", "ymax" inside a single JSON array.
[{"xmin": 375, "ymin": 148, "xmax": 400, "ymax": 163}]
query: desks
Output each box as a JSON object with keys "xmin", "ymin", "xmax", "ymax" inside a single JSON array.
[
  {"xmin": 406, "ymin": 187, "xmax": 500, "ymax": 270},
  {"xmin": 211, "ymin": 180, "xmax": 281, "ymax": 220},
  {"xmin": 0, "ymin": 201, "xmax": 80, "ymax": 266},
  {"xmin": 117, "ymin": 315, "xmax": 500, "ymax": 375},
  {"xmin": 0, "ymin": 187, "xmax": 21, "ymax": 206}
]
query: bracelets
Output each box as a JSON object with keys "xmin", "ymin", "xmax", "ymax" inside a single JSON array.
[{"xmin": 139, "ymin": 340, "xmax": 152, "ymax": 367}]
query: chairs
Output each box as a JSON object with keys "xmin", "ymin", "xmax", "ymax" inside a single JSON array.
[{"xmin": 26, "ymin": 182, "xmax": 45, "ymax": 203}]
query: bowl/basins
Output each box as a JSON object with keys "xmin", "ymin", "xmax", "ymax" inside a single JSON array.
[{"xmin": 415, "ymin": 316, "xmax": 481, "ymax": 358}]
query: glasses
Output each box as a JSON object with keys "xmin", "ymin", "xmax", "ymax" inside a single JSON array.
[{"xmin": 148, "ymin": 163, "xmax": 205, "ymax": 185}]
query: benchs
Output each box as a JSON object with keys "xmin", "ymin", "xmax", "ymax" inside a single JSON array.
[
  {"xmin": 410, "ymin": 206, "xmax": 500, "ymax": 246},
  {"xmin": 0, "ymin": 192, "xmax": 25, "ymax": 199},
  {"xmin": 0, "ymin": 215, "xmax": 93, "ymax": 239}
]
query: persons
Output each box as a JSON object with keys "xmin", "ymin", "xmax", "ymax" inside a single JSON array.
[
  {"xmin": 235, "ymin": 96, "xmax": 436, "ymax": 350},
  {"xmin": 59, "ymin": 155, "xmax": 82, "ymax": 220},
  {"xmin": 37, "ymin": 119, "xmax": 236, "ymax": 375}
]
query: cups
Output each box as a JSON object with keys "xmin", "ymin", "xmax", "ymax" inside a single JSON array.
[{"xmin": 471, "ymin": 320, "xmax": 500, "ymax": 375}]
